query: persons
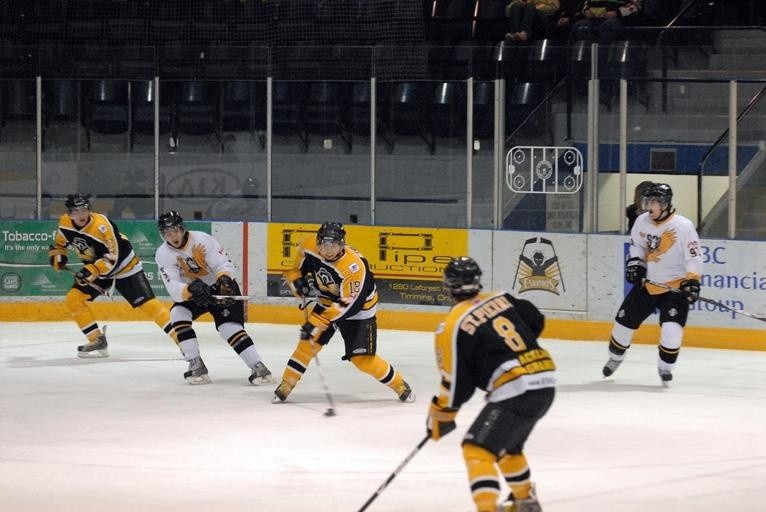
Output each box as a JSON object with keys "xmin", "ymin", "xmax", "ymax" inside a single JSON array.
[
  {"xmin": 626, "ymin": 182, "xmax": 654, "ymax": 231},
  {"xmin": 426, "ymin": 256, "xmax": 557, "ymax": 511},
  {"xmin": 500, "ymin": 0, "xmax": 559, "ymax": 42},
  {"xmin": 603, "ymin": 183, "xmax": 704, "ymax": 380},
  {"xmin": 275, "ymin": 221, "xmax": 412, "ymax": 402},
  {"xmin": 50, "ymin": 193, "xmax": 182, "ymax": 357},
  {"xmin": 153, "ymin": 211, "xmax": 271, "ymax": 382},
  {"xmin": 560, "ymin": 0, "xmax": 647, "ymax": 41}
]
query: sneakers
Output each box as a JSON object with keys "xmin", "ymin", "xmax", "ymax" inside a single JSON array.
[
  {"xmin": 603, "ymin": 357, "xmax": 623, "ymax": 376},
  {"xmin": 659, "ymin": 368, "xmax": 672, "ymax": 382},
  {"xmin": 274, "ymin": 380, "xmax": 295, "ymax": 401},
  {"xmin": 184, "ymin": 356, "xmax": 208, "ymax": 379},
  {"xmin": 248, "ymin": 361, "xmax": 271, "ymax": 383},
  {"xmin": 78, "ymin": 324, "xmax": 107, "ymax": 352},
  {"xmin": 495, "ymin": 481, "xmax": 541, "ymax": 511},
  {"xmin": 396, "ymin": 379, "xmax": 411, "ymax": 402}
]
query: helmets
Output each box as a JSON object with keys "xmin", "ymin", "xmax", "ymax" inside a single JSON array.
[
  {"xmin": 640, "ymin": 183, "xmax": 673, "ymax": 210},
  {"xmin": 316, "ymin": 221, "xmax": 346, "ymax": 254},
  {"xmin": 636, "ymin": 181, "xmax": 657, "ymax": 209},
  {"xmin": 158, "ymin": 210, "xmax": 185, "ymax": 243},
  {"xmin": 444, "ymin": 256, "xmax": 483, "ymax": 297},
  {"xmin": 65, "ymin": 193, "xmax": 93, "ymax": 219}
]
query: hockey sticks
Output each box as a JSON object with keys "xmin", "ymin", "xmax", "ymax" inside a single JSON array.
[
  {"xmin": 300, "ymin": 293, "xmax": 336, "ymax": 418},
  {"xmin": 48, "ymin": 244, "xmax": 116, "ymax": 300}
]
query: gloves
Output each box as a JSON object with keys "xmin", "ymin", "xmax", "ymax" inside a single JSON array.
[
  {"xmin": 213, "ymin": 275, "xmax": 237, "ymax": 308},
  {"xmin": 427, "ymin": 395, "xmax": 457, "ymax": 441},
  {"xmin": 678, "ymin": 279, "xmax": 700, "ymax": 304},
  {"xmin": 625, "ymin": 257, "xmax": 648, "ymax": 290},
  {"xmin": 48, "ymin": 249, "xmax": 69, "ymax": 272},
  {"xmin": 187, "ymin": 278, "xmax": 217, "ymax": 308},
  {"xmin": 300, "ymin": 315, "xmax": 328, "ymax": 339},
  {"xmin": 74, "ymin": 265, "xmax": 100, "ymax": 286},
  {"xmin": 283, "ymin": 268, "xmax": 310, "ymax": 296}
]
